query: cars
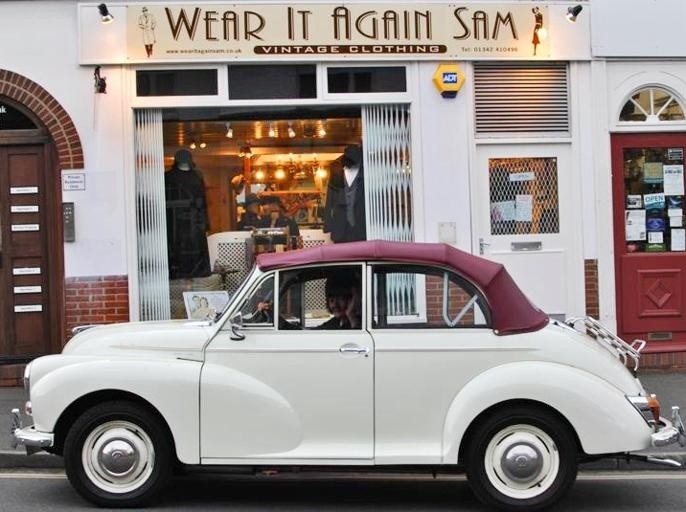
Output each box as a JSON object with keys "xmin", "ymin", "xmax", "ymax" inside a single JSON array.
[{"xmin": 11, "ymin": 239, "xmax": 686, "ymax": 511}]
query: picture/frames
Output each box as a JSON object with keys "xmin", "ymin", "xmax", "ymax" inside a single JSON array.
[{"xmin": 182, "ymin": 290, "xmax": 229, "ymax": 320}]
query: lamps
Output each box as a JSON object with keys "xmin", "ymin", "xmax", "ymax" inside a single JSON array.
[
  {"xmin": 566, "ymin": 5, "xmax": 584, "ymax": 22},
  {"xmin": 98, "ymin": 4, "xmax": 113, "ymax": 24}
]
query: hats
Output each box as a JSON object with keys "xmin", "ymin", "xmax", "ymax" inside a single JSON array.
[
  {"xmin": 343, "ymin": 145, "xmax": 361, "ymax": 165},
  {"xmin": 172, "ymin": 149, "xmax": 196, "ymax": 168},
  {"xmin": 243, "ymin": 193, "xmax": 261, "ymax": 210}
]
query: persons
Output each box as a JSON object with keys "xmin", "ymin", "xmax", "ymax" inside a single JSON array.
[
  {"xmin": 236, "ymin": 192, "xmax": 262, "ymax": 232},
  {"xmin": 531, "ymin": 6, "xmax": 543, "ymax": 55},
  {"xmin": 259, "ymin": 194, "xmax": 299, "ymax": 240},
  {"xmin": 256, "ymin": 267, "xmax": 362, "ymax": 330},
  {"xmin": 191, "ymin": 295, "xmax": 213, "ymax": 319},
  {"xmin": 164, "ymin": 149, "xmax": 212, "ymax": 279},
  {"xmin": 138, "ymin": 7, "xmax": 156, "ymax": 57},
  {"xmin": 322, "ymin": 142, "xmax": 367, "ymax": 243}
]
